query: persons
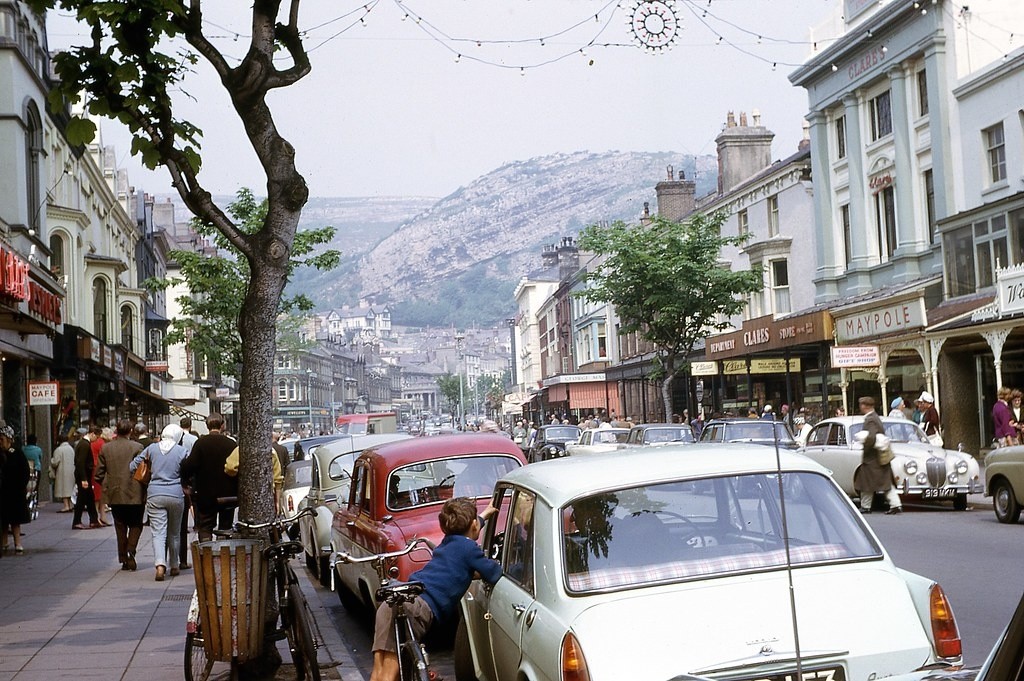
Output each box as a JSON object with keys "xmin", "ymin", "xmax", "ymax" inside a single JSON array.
[
  {"xmin": 225, "ymin": 432, "xmax": 290, "ymax": 516},
  {"xmin": 130, "ymin": 418, "xmax": 199, "ymax": 581},
  {"xmin": 181, "ymin": 412, "xmax": 238, "ymax": 542},
  {"xmin": 748, "ymin": 407, "xmax": 759, "ymax": 418},
  {"xmin": 440, "ymin": 419, "xmax": 499, "ymax": 433},
  {"xmin": 684, "ymin": 409, "xmax": 720, "ymax": 438},
  {"xmin": 52, "ymin": 419, "xmax": 157, "ymax": 571},
  {"xmin": 912, "ymin": 392, "xmax": 940, "ymax": 435},
  {"xmin": 993, "ymin": 387, "xmax": 1024, "ymax": 447},
  {"xmin": 22, "ymin": 434, "xmax": 43, "ymax": 492},
  {"xmin": 889, "ymin": 397, "xmax": 907, "ymax": 437},
  {"xmin": 794, "ymin": 413, "xmax": 818, "ymax": 442},
  {"xmin": 781, "ymin": 405, "xmax": 789, "ymax": 424},
  {"xmin": 0, "ymin": 426, "xmax": 32, "ymax": 555},
  {"xmin": 278, "ymin": 431, "xmax": 300, "ymax": 441},
  {"xmin": 578, "ymin": 409, "xmax": 637, "ymax": 442},
  {"xmin": 859, "ymin": 397, "xmax": 903, "ymax": 514},
  {"xmin": 513, "ymin": 413, "xmax": 569, "ymax": 456},
  {"xmin": 762, "ymin": 403, "xmax": 776, "ymax": 420},
  {"xmin": 370, "ymin": 496, "xmax": 503, "ymax": 681},
  {"xmin": 507, "ymin": 495, "xmax": 623, "ymax": 583},
  {"xmin": 829, "ymin": 407, "xmax": 845, "ymax": 441}
]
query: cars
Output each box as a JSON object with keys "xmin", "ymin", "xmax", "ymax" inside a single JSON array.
[
  {"xmin": 455, "ymin": 441, "xmax": 965, "ymax": 681},
  {"xmin": 281, "ymin": 409, "xmax": 578, "ymax": 651},
  {"xmin": 525, "ymin": 419, "xmax": 800, "ymax": 500},
  {"xmin": 785, "ymin": 416, "xmax": 985, "ymax": 512},
  {"xmin": 983, "ymin": 445, "xmax": 1023, "ymax": 523}
]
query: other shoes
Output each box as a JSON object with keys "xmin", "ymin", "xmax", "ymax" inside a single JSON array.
[
  {"xmin": 55, "ymin": 503, "xmax": 113, "ymax": 530},
  {"xmin": 126, "ymin": 552, "xmax": 136, "ymax": 571},
  {"xmin": 170, "ymin": 567, "xmax": 180, "ymax": 577},
  {"xmin": 179, "ymin": 563, "xmax": 192, "ymax": 569},
  {"xmin": 8, "ymin": 530, "xmax": 26, "ymax": 536},
  {"xmin": 884, "ymin": 507, "xmax": 901, "ymax": 515},
  {"xmin": 15, "ymin": 546, "xmax": 24, "ymax": 555},
  {"xmin": 143, "ymin": 521, "xmax": 150, "ymax": 526},
  {"xmin": 155, "ymin": 569, "xmax": 165, "ymax": 580},
  {"xmin": 187, "ymin": 530, "xmax": 190, "ymax": 533},
  {"xmin": 121, "ymin": 561, "xmax": 130, "ymax": 571},
  {"xmin": 858, "ymin": 508, "xmax": 871, "ymax": 513},
  {"xmin": 0, "ymin": 543, "xmax": 9, "ymax": 552}
]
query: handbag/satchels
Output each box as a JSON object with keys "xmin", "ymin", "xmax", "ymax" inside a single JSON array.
[
  {"xmin": 133, "ymin": 449, "xmax": 151, "ymax": 485},
  {"xmin": 877, "ymin": 446, "xmax": 895, "ymax": 465},
  {"xmin": 920, "ymin": 421, "xmax": 943, "ymax": 447},
  {"xmin": 514, "ymin": 435, "xmax": 523, "ymax": 444},
  {"xmin": 526, "ymin": 438, "xmax": 534, "ymax": 448}
]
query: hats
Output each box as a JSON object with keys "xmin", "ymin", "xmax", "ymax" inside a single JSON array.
[
  {"xmin": 922, "ymin": 391, "xmax": 934, "ymax": 403},
  {"xmin": 914, "ymin": 395, "xmax": 923, "ymax": 406},
  {"xmin": 0, "ymin": 426, "xmax": 14, "ymax": 439},
  {"xmin": 860, "ymin": 397, "xmax": 874, "ymax": 405},
  {"xmin": 794, "ymin": 417, "xmax": 805, "ymax": 424},
  {"xmin": 783, "ymin": 405, "xmax": 789, "ymax": 410},
  {"xmin": 891, "ymin": 397, "xmax": 902, "ymax": 409}
]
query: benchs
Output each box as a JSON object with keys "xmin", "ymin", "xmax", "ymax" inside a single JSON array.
[{"xmin": 567, "ymin": 543, "xmax": 850, "ymax": 592}]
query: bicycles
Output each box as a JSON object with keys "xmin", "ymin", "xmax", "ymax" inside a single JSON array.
[
  {"xmin": 329, "ymin": 537, "xmax": 437, "ymax": 681},
  {"xmin": 182, "ymin": 496, "xmax": 238, "ymax": 681},
  {"xmin": 231, "ymin": 506, "xmax": 321, "ymax": 681}
]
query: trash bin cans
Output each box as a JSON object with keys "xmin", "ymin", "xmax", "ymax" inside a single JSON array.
[{"xmin": 190, "ymin": 535, "xmax": 271, "ymax": 664}]
